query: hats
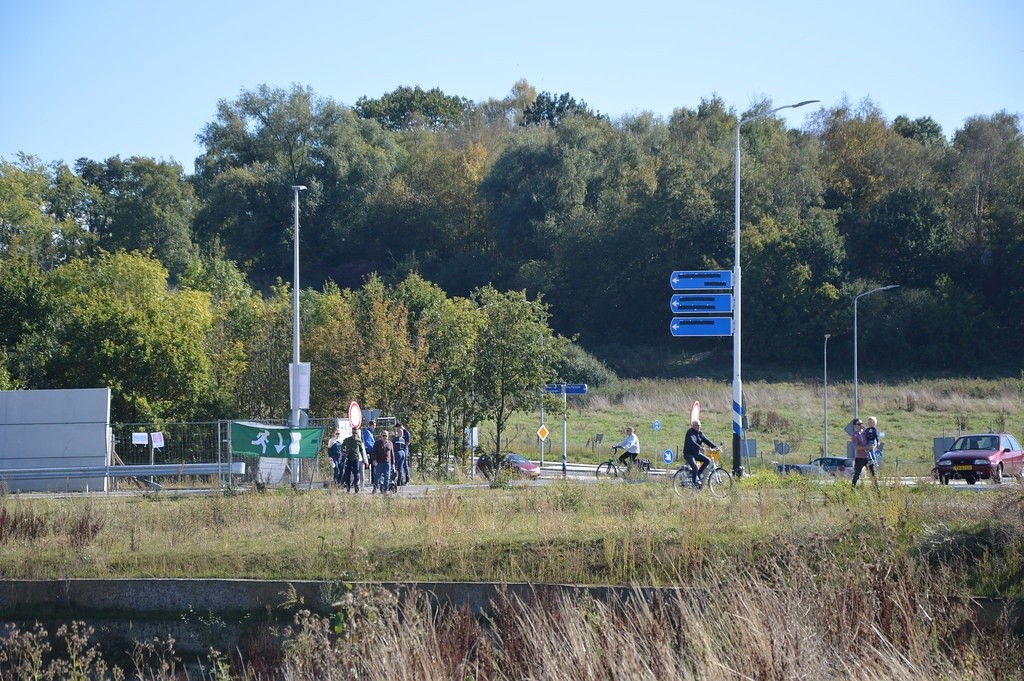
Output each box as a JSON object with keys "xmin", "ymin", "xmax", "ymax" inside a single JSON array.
[{"xmin": 392, "ymin": 423, "xmax": 401, "ymax": 428}]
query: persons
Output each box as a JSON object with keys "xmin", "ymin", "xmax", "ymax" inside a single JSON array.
[
  {"xmin": 356, "ymin": 435, "xmax": 369, "ymax": 491},
  {"xmin": 392, "ymin": 428, "xmax": 406, "ymax": 485},
  {"xmin": 990, "ymin": 437, "xmax": 998, "ymax": 450},
  {"xmin": 851, "ymin": 419, "xmax": 878, "ymax": 489},
  {"xmin": 341, "ymin": 429, "xmax": 369, "ymax": 492},
  {"xmin": 862, "ymin": 416, "xmax": 880, "ymax": 466},
  {"xmin": 328, "ymin": 430, "xmax": 342, "ymax": 487},
  {"xmin": 364, "ymin": 420, "xmax": 376, "ymax": 464},
  {"xmin": 371, "ymin": 430, "xmax": 396, "ymax": 494},
  {"xmin": 395, "ymin": 423, "xmax": 411, "ymax": 485},
  {"xmin": 683, "ymin": 420, "xmax": 722, "ymax": 492},
  {"xmin": 616, "ymin": 427, "xmax": 639, "ymax": 466}
]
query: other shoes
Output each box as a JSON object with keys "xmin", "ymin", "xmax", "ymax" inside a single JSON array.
[
  {"xmin": 347, "ymin": 487, "xmax": 350, "ymax": 492},
  {"xmin": 355, "ymin": 486, "xmax": 359, "ymax": 493},
  {"xmin": 697, "ymin": 473, "xmax": 703, "ymax": 479},
  {"xmin": 397, "ymin": 480, "xmax": 404, "ymax": 486},
  {"xmin": 405, "ymin": 481, "xmax": 410, "ymax": 485},
  {"xmin": 372, "ymin": 488, "xmax": 377, "ymax": 493}
]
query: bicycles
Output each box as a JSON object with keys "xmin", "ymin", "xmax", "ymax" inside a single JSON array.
[
  {"xmin": 596, "ymin": 446, "xmax": 650, "ymax": 481},
  {"xmin": 672, "ymin": 447, "xmax": 732, "ymax": 498}
]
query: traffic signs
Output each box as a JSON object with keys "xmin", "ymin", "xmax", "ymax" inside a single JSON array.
[
  {"xmin": 669, "ymin": 317, "xmax": 732, "ymax": 338},
  {"xmin": 565, "ymin": 383, "xmax": 589, "ymax": 396},
  {"xmin": 671, "ymin": 270, "xmax": 733, "ymax": 289},
  {"xmin": 541, "ymin": 384, "xmax": 563, "ymax": 398},
  {"xmin": 670, "ymin": 294, "xmax": 732, "ymax": 314}
]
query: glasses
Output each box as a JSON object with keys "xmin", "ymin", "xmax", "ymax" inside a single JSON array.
[
  {"xmin": 382, "ymin": 435, "xmax": 387, "ymax": 437},
  {"xmin": 856, "ymin": 423, "xmax": 863, "ymax": 425},
  {"xmin": 353, "ymin": 433, "xmax": 358, "ymax": 434},
  {"xmin": 335, "ymin": 433, "xmax": 340, "ymax": 435}
]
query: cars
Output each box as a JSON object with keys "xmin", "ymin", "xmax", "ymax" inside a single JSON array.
[
  {"xmin": 809, "ymin": 456, "xmax": 867, "ymax": 478},
  {"xmin": 504, "ymin": 453, "xmax": 541, "ymax": 481},
  {"xmin": 936, "ymin": 434, "xmax": 1024, "ymax": 485}
]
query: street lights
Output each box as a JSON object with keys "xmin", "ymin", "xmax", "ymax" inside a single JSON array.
[
  {"xmin": 291, "ymin": 186, "xmax": 308, "ymax": 487},
  {"xmin": 853, "ymin": 284, "xmax": 902, "ymax": 420},
  {"xmin": 732, "ymin": 99, "xmax": 822, "ymax": 478}
]
